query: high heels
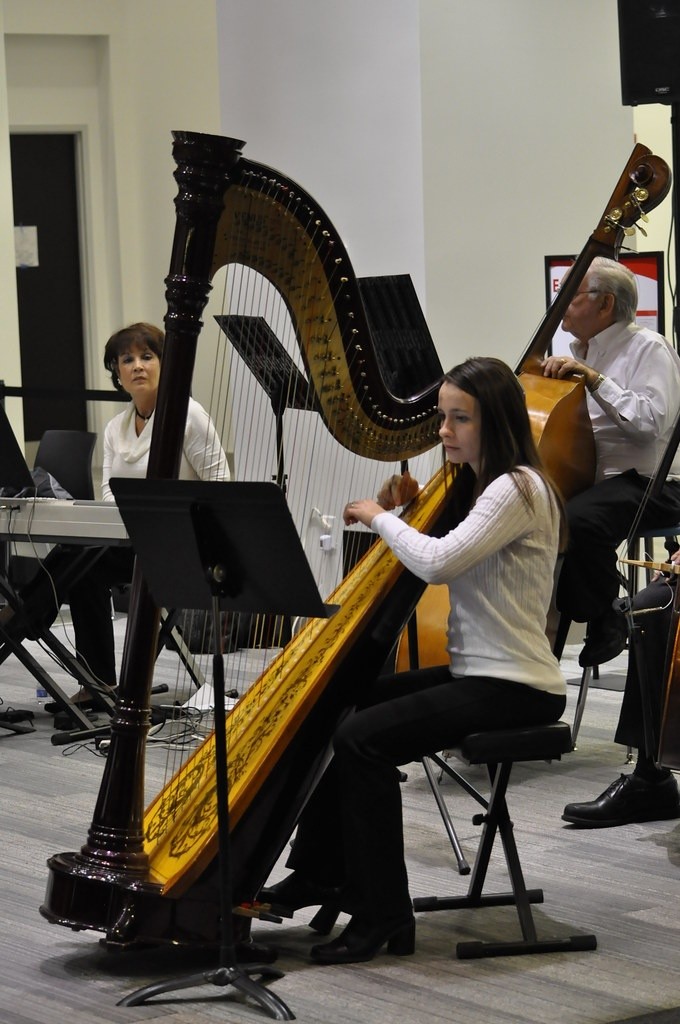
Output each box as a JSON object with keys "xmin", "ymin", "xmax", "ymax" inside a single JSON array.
[
  {"xmin": 255, "ymin": 868, "xmax": 341, "ymax": 934},
  {"xmin": 310, "ymin": 903, "xmax": 416, "ymax": 966}
]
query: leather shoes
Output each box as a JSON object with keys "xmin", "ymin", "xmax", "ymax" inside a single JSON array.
[{"xmin": 562, "ymin": 773, "xmax": 680, "ymax": 825}]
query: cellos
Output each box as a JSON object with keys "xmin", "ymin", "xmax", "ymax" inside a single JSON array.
[{"xmin": 329, "ymin": 138, "xmax": 675, "ymax": 790}]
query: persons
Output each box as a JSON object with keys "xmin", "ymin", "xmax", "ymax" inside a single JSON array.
[
  {"xmin": 537, "ymin": 255, "xmax": 679, "ymax": 669},
  {"xmin": 560, "ymin": 551, "xmax": 679, "ymax": 827},
  {"xmin": 0, "ymin": 322, "xmax": 233, "ymax": 713},
  {"xmin": 258, "ymin": 356, "xmax": 569, "ymax": 964}
]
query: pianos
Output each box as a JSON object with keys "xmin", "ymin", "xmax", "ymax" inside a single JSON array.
[{"xmin": 1, "ymin": 495, "xmax": 133, "ymax": 549}]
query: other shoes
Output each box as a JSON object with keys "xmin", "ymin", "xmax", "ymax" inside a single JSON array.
[
  {"xmin": 44, "ymin": 680, "xmax": 116, "ymax": 713},
  {"xmin": 579, "ymin": 618, "xmax": 630, "ymax": 667}
]
